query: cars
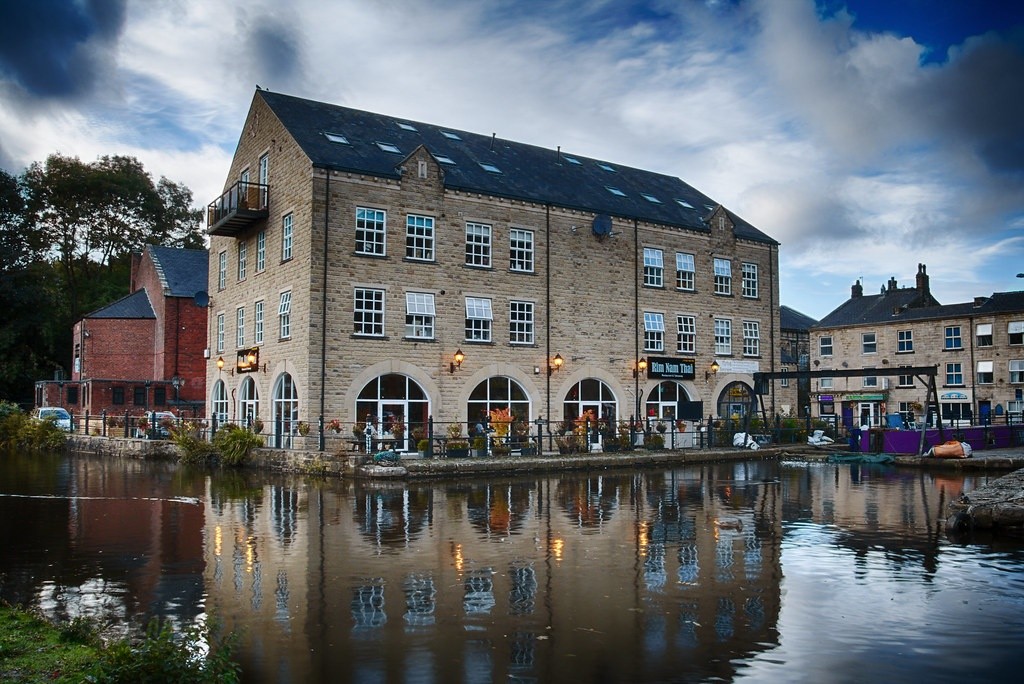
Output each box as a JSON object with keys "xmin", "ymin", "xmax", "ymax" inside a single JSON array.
[
  {"xmin": 134, "ymin": 410, "xmax": 179, "ymax": 440},
  {"xmin": 31, "ymin": 406, "xmax": 75, "ymax": 433}
]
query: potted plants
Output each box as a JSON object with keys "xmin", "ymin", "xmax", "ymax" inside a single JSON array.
[
  {"xmin": 295, "ymin": 422, "xmax": 310, "ymax": 437},
  {"xmin": 445, "ymin": 442, "xmax": 469, "ymax": 457},
  {"xmin": 784, "ymin": 420, "xmax": 798, "ymax": 443},
  {"xmin": 553, "ymin": 420, "xmax": 668, "ymax": 454},
  {"xmin": 474, "ymin": 436, "xmax": 487, "ymax": 458},
  {"xmin": 390, "ymin": 424, "xmax": 406, "ymax": 439},
  {"xmin": 677, "ymin": 423, "xmax": 686, "ymax": 432},
  {"xmin": 354, "ymin": 424, "xmax": 365, "ymax": 440},
  {"xmin": 492, "ymin": 446, "xmax": 512, "ymax": 457},
  {"xmin": 520, "ymin": 444, "xmax": 536, "ymax": 455},
  {"xmin": 417, "ymin": 439, "xmax": 429, "ymax": 458},
  {"xmin": 514, "ymin": 423, "xmax": 529, "ymax": 435},
  {"xmin": 251, "ymin": 421, "xmax": 264, "ymax": 434},
  {"xmin": 451, "ymin": 428, "xmax": 460, "ymax": 438},
  {"xmin": 325, "ymin": 419, "xmax": 343, "ymax": 435}
]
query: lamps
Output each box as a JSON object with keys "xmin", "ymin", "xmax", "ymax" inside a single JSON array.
[
  {"xmin": 248, "ymin": 350, "xmax": 266, "ymax": 373},
  {"xmin": 450, "ymin": 349, "xmax": 465, "ymax": 373},
  {"xmin": 633, "ymin": 357, "xmax": 647, "ymax": 378},
  {"xmin": 705, "ymin": 360, "xmax": 720, "ymax": 381},
  {"xmin": 548, "ymin": 353, "xmax": 563, "ymax": 376},
  {"xmin": 217, "ymin": 356, "xmax": 234, "ymax": 377}
]
